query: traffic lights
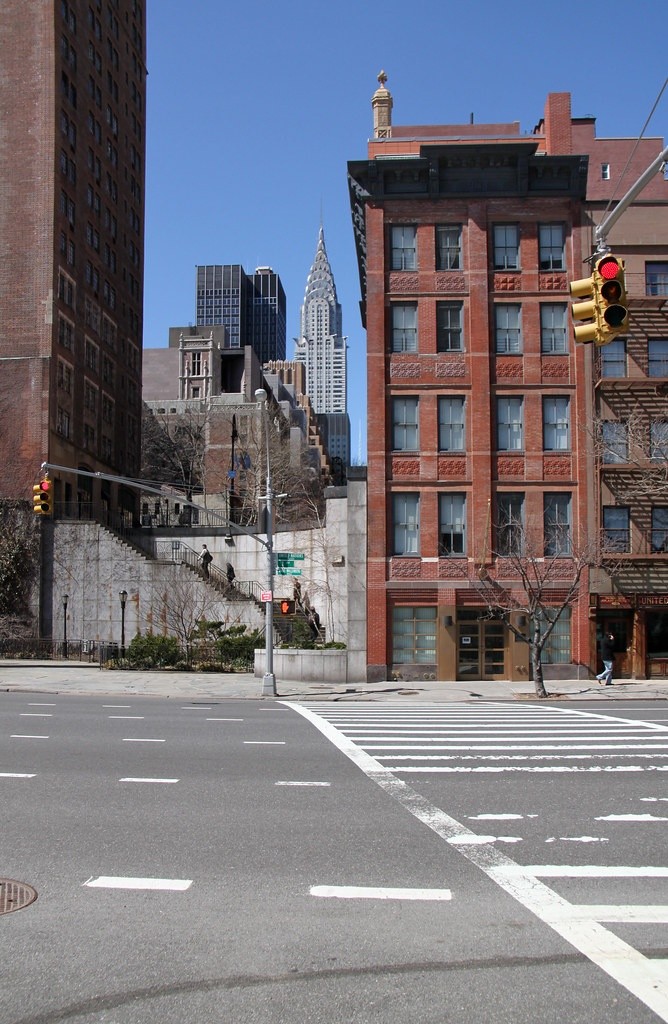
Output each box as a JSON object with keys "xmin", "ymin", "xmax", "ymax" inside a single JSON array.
[
  {"xmin": 280, "ymin": 598, "xmax": 297, "ymax": 617},
  {"xmin": 39, "ymin": 479, "xmax": 53, "ymax": 514},
  {"xmin": 33, "ymin": 484, "xmax": 46, "ymax": 515},
  {"xmin": 570, "ymin": 271, "xmax": 615, "ymax": 346},
  {"xmin": 594, "ymin": 253, "xmax": 631, "ymax": 335}
]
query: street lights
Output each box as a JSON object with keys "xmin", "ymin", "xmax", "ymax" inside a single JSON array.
[
  {"xmin": 254, "ymin": 388, "xmax": 279, "ymax": 699},
  {"xmin": 328, "ymin": 456, "xmax": 344, "ymax": 487},
  {"xmin": 164, "ymin": 498, "xmax": 169, "ymax": 527},
  {"xmin": 228, "ymin": 413, "xmax": 240, "ymax": 527}
]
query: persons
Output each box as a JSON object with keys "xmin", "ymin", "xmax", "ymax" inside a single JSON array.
[
  {"xmin": 294, "ymin": 578, "xmax": 303, "ymax": 611},
  {"xmin": 310, "ymin": 607, "xmax": 320, "ymax": 637},
  {"xmin": 198, "ymin": 544, "xmax": 213, "ymax": 579},
  {"xmin": 595, "ymin": 632, "xmax": 615, "ymax": 685},
  {"xmin": 226, "ymin": 563, "xmax": 236, "ymax": 590}
]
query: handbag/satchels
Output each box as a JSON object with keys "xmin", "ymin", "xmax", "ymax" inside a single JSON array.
[{"xmin": 203, "ymin": 550, "xmax": 213, "ymax": 563}]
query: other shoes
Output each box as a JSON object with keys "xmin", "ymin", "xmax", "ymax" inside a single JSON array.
[
  {"xmin": 606, "ymin": 683, "xmax": 614, "ymax": 685},
  {"xmin": 596, "ymin": 675, "xmax": 601, "ymax": 684}
]
